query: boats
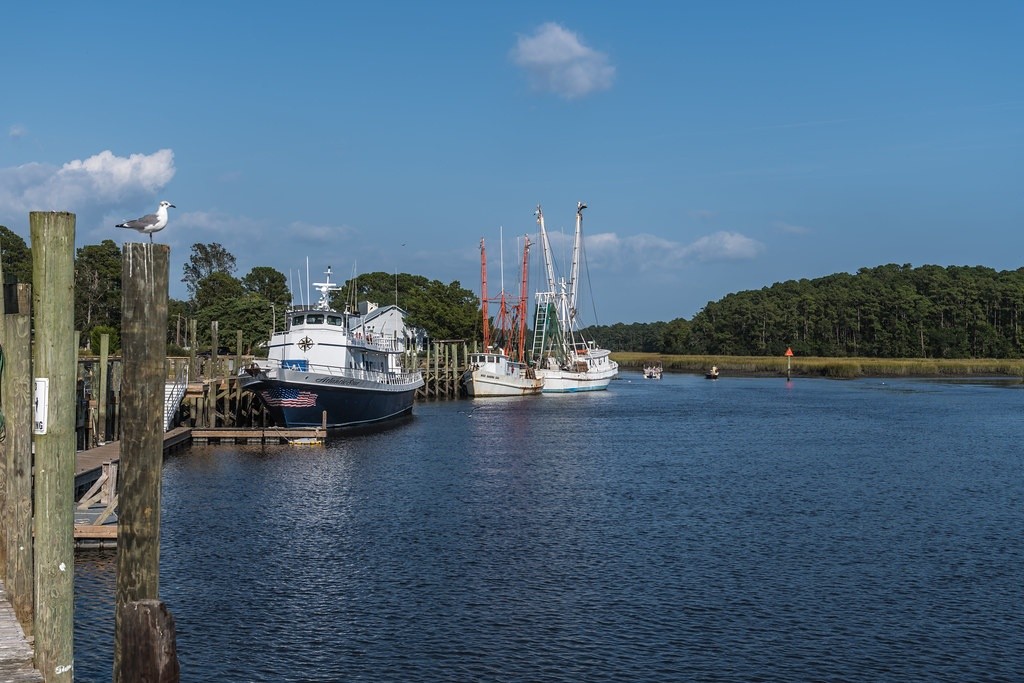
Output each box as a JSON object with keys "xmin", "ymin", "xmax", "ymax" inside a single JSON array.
[
  {"xmin": 236, "ymin": 256, "xmax": 425, "ymax": 437},
  {"xmin": 642, "ymin": 359, "xmax": 663, "ymax": 380},
  {"xmin": 463, "ymin": 353, "xmax": 544, "ymax": 395},
  {"xmin": 705, "ymin": 372, "xmax": 719, "ymax": 379},
  {"xmin": 531, "ymin": 278, "xmax": 618, "ymax": 392}
]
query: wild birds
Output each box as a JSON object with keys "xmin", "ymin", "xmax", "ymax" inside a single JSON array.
[{"xmin": 115, "ymin": 201, "xmax": 176, "ymax": 244}]
